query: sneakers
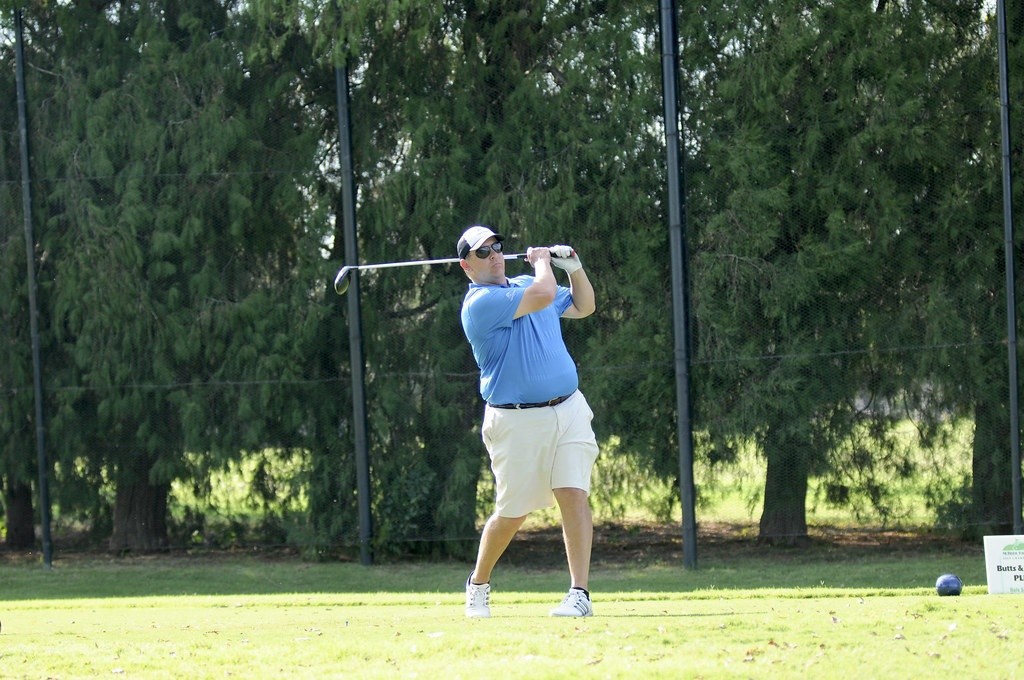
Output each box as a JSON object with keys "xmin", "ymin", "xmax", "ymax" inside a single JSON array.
[
  {"xmin": 466, "ymin": 571, "xmax": 491, "ymax": 618},
  {"xmin": 552, "ymin": 589, "xmax": 593, "ymax": 618}
]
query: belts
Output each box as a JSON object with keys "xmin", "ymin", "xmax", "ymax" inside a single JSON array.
[{"xmin": 489, "ymin": 394, "xmax": 571, "ymax": 408}]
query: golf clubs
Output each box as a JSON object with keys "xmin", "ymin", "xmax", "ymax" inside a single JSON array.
[{"xmin": 334, "ymin": 249, "xmax": 575, "ymax": 295}]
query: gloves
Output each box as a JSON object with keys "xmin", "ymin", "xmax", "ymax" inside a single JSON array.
[{"xmin": 550, "ymin": 245, "xmax": 582, "ymax": 275}]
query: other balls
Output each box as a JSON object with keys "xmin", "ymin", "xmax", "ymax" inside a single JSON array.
[{"xmin": 936, "ymin": 573, "xmax": 962, "ymax": 597}]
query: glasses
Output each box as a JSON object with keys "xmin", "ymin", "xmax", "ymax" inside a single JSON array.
[{"xmin": 476, "ymin": 242, "xmax": 503, "ymax": 259}]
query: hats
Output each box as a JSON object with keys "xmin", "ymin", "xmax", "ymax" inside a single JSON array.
[{"xmin": 457, "ymin": 225, "xmax": 505, "ymax": 261}]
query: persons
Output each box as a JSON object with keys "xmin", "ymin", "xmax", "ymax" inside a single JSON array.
[{"xmin": 456, "ymin": 226, "xmax": 600, "ymax": 618}]
